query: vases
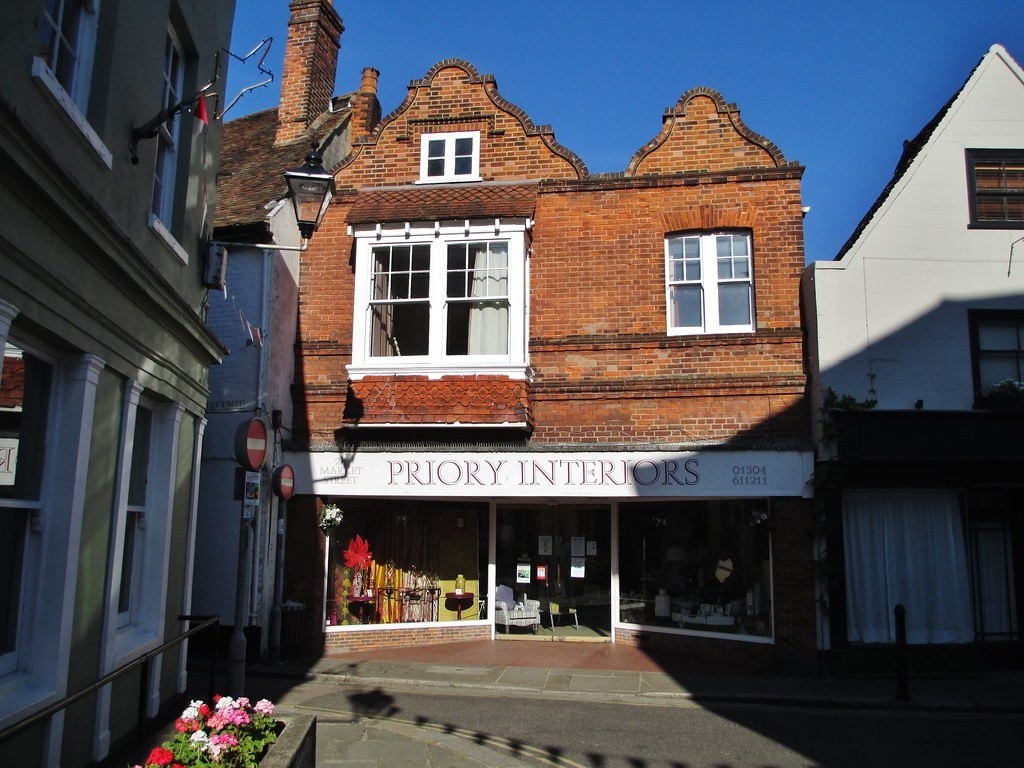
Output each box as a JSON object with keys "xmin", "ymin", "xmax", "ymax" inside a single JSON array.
[{"xmin": 88, "ymin": 710, "xmax": 318, "ymax": 768}]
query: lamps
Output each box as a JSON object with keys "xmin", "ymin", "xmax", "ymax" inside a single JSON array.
[{"xmin": 201, "ymin": 136, "xmax": 337, "ymax": 290}]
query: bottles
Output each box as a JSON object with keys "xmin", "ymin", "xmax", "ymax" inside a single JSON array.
[{"xmin": 456, "ymin": 574, "xmax": 465, "ymax": 596}]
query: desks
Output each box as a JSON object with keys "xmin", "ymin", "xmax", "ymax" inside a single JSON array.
[
  {"xmin": 447, "ymin": 593, "xmax": 474, "ymax": 617},
  {"xmin": 346, "ymin": 594, "xmax": 376, "ymax": 623}
]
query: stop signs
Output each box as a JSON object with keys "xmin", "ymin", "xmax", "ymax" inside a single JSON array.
[
  {"xmin": 280, "ymin": 465, "xmax": 296, "ymax": 500},
  {"xmin": 245, "ymin": 420, "xmax": 266, "ymax": 471}
]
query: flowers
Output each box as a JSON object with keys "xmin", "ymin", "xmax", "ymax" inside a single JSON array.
[{"xmin": 128, "ymin": 694, "xmax": 280, "ymax": 768}]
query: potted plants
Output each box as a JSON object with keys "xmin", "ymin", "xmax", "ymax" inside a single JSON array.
[{"xmin": 318, "ymin": 503, "xmax": 344, "ymax": 530}]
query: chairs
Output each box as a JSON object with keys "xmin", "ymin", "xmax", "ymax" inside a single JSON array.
[{"xmin": 496, "ymin": 585, "xmax": 540, "ymax": 634}]
